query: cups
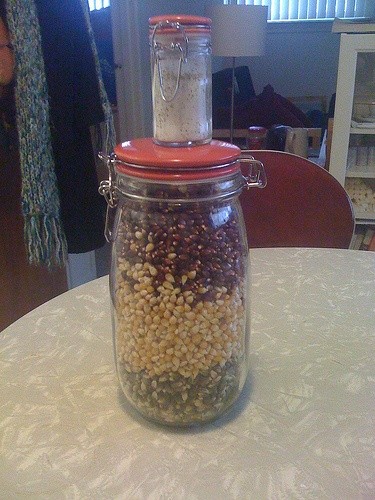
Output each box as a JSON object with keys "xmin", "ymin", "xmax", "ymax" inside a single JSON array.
[
  {"xmin": 306, "ymin": 127, "xmax": 322, "ymax": 158},
  {"xmin": 283, "ymin": 128, "xmax": 309, "ymax": 160}
]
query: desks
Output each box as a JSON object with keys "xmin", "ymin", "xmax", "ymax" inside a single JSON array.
[{"xmin": 0, "ymin": 247, "xmax": 375, "ymax": 500}]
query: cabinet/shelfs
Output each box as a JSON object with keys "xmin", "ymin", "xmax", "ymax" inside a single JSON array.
[{"xmin": 328, "ymin": 33, "xmax": 375, "ymax": 225}]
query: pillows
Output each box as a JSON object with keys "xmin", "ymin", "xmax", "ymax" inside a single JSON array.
[{"xmin": 214, "ymin": 84, "xmax": 307, "ymax": 128}]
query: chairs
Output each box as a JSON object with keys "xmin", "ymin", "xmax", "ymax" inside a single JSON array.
[{"xmin": 238, "ymin": 149, "xmax": 356, "ymax": 250}]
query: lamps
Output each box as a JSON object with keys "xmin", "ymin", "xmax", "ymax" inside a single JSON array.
[{"xmin": 204, "ymin": 4, "xmax": 269, "ymax": 143}]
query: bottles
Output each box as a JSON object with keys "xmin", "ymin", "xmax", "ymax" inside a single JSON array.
[
  {"xmin": 97, "ymin": 135, "xmax": 268, "ymax": 428},
  {"xmin": 148, "ymin": 15, "xmax": 213, "ymax": 147}
]
